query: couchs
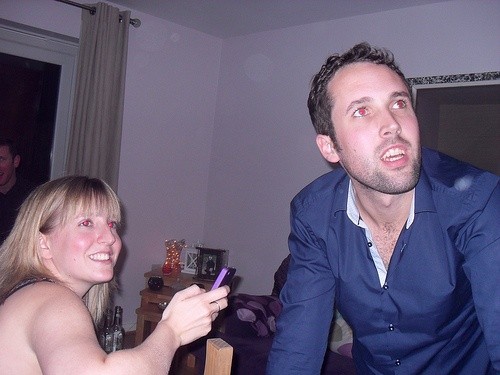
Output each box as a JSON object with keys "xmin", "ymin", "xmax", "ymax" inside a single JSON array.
[{"xmin": 187, "ymin": 254, "xmax": 355, "ymax": 375}]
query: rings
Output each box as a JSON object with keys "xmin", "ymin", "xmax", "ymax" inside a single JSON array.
[{"xmin": 213, "ymin": 301, "xmax": 221, "ymax": 313}]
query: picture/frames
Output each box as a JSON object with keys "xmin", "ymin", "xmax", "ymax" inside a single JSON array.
[{"xmin": 194, "ymin": 246, "xmax": 226, "ymax": 282}]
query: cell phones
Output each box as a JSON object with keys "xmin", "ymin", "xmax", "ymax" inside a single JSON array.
[{"xmin": 211, "ymin": 267, "xmax": 236, "ymax": 291}]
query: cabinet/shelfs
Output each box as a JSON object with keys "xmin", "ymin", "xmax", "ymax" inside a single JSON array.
[{"xmin": 134, "ymin": 269, "xmax": 237, "ymax": 348}]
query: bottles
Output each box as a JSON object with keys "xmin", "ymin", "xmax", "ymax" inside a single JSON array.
[
  {"xmin": 98, "ymin": 310, "xmax": 113, "ymax": 355},
  {"xmin": 112, "ymin": 305, "xmax": 124, "ymax": 352}
]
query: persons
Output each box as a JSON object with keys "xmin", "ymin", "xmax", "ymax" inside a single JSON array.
[
  {"xmin": 0, "ymin": 175, "xmax": 229, "ymax": 375},
  {"xmin": 265, "ymin": 41, "xmax": 500, "ymax": 375},
  {"xmin": 0, "ymin": 139, "xmax": 39, "ymax": 245},
  {"xmin": 204, "ymin": 255, "xmax": 215, "ymax": 275}
]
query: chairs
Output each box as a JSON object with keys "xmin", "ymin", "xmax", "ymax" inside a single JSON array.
[{"xmin": 203, "ymin": 338, "xmax": 233, "ymax": 375}]
